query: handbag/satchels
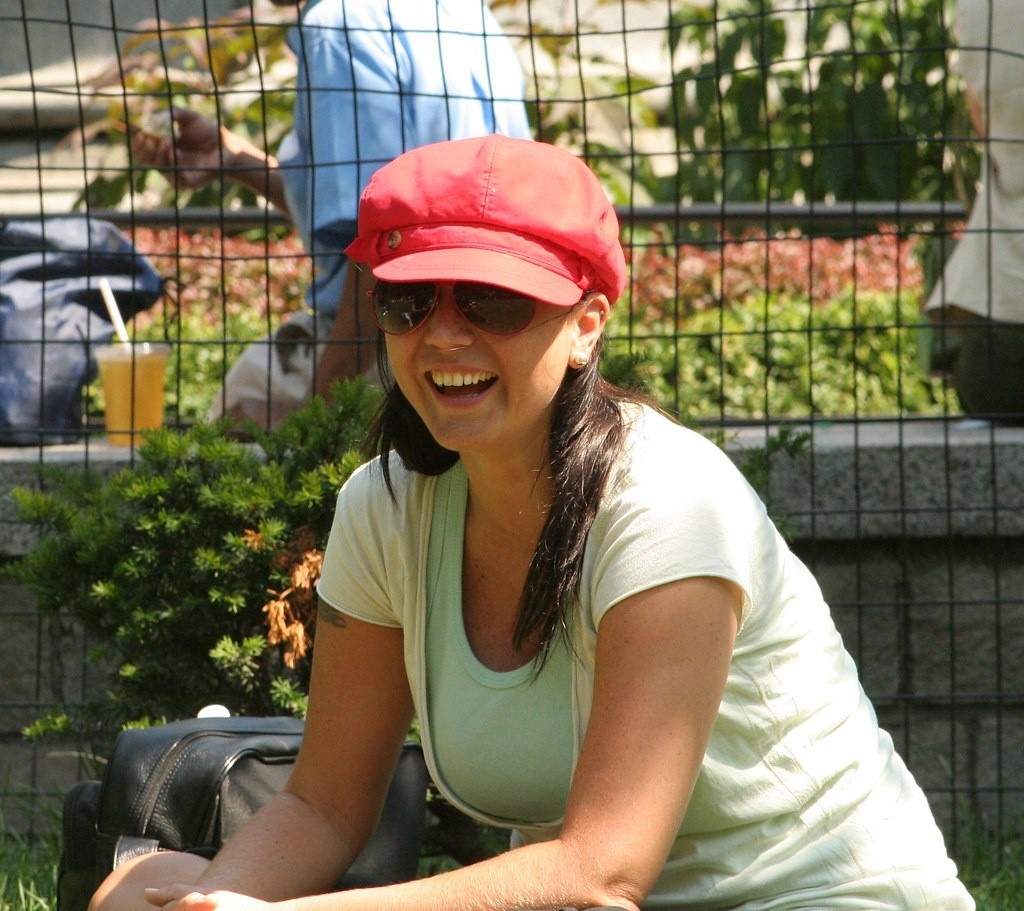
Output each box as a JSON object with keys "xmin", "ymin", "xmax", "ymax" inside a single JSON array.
[
  {"xmin": 0, "ymin": 217, "xmax": 163, "ymax": 445},
  {"xmin": 93, "ymin": 715, "xmax": 430, "ymax": 892}
]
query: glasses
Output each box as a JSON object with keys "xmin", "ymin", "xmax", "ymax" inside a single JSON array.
[{"xmin": 366, "ymin": 282, "xmax": 540, "ymax": 336}]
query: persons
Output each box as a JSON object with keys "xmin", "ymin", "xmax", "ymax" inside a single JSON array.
[
  {"xmin": 115, "ymin": 0, "xmax": 539, "ymax": 446},
  {"xmin": 924, "ymin": 0, "xmax": 1024, "ymax": 427},
  {"xmin": 88, "ymin": 135, "xmax": 975, "ymax": 911}
]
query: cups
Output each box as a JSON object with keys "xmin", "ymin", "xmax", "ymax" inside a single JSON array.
[{"xmin": 92, "ymin": 340, "xmax": 166, "ymax": 443}]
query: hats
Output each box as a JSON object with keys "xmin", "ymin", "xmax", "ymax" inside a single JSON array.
[{"xmin": 342, "ymin": 135, "xmax": 627, "ymax": 305}]
